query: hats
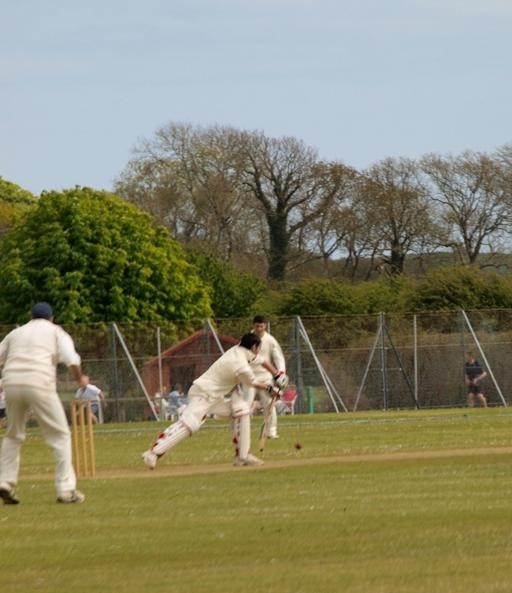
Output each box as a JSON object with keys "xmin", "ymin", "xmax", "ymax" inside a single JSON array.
[{"xmin": 31, "ymin": 302, "xmax": 53, "ymax": 317}]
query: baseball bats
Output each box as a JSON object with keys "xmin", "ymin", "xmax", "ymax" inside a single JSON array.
[{"xmin": 258, "ymin": 393, "xmax": 276, "ymax": 453}]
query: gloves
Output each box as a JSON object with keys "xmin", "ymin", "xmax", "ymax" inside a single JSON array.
[{"xmin": 265, "ymin": 370, "xmax": 289, "ymax": 401}]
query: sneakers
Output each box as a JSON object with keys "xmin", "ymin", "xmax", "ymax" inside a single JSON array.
[
  {"xmin": 0, "ymin": 481, "xmax": 19, "ymax": 504},
  {"xmin": 234, "ymin": 454, "xmax": 263, "ymax": 466},
  {"xmin": 56, "ymin": 490, "xmax": 86, "ymax": 503},
  {"xmin": 140, "ymin": 449, "xmax": 158, "ymax": 469}
]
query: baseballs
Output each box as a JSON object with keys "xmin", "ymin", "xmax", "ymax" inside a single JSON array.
[{"xmin": 295, "ymin": 441, "xmax": 303, "ymax": 449}]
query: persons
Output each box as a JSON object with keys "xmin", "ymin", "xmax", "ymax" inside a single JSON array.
[
  {"xmin": 140, "ymin": 315, "xmax": 295, "ymax": 472},
  {"xmin": 73, "ymin": 374, "xmax": 107, "ymax": 423},
  {"xmin": 0, "ymin": 302, "xmax": 88, "ymax": 504},
  {"xmin": 464, "ymin": 351, "xmax": 489, "ymax": 407}
]
